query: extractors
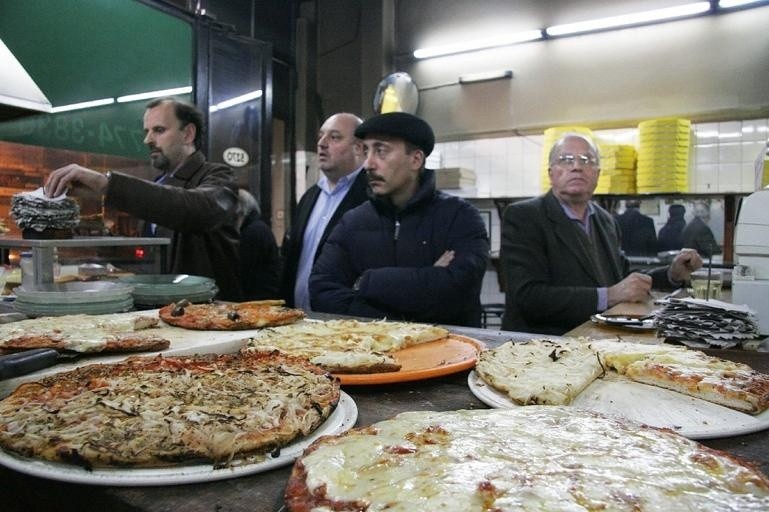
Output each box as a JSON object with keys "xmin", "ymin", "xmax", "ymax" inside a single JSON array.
[{"xmin": 1, "ymin": 34, "xmax": 53, "ymax": 120}]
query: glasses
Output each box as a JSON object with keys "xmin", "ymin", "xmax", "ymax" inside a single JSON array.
[{"xmin": 550, "ymin": 153, "xmax": 599, "ymax": 166}]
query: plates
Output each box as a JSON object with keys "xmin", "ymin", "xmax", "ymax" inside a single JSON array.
[
  {"xmin": 11, "ymin": 282, "xmax": 135, "ymax": 319},
  {"xmin": 1, "ymin": 388, "xmax": 362, "ymax": 489},
  {"xmin": 240, "ymin": 332, "xmax": 488, "ymax": 387},
  {"xmin": 121, "ymin": 274, "xmax": 219, "ymax": 305},
  {"xmin": 466, "ymin": 337, "xmax": 769, "ymax": 441}
]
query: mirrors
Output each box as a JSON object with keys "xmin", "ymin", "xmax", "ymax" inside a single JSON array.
[
  {"xmin": 117, "ymin": 86, "xmax": 193, "ymax": 104},
  {"xmin": 210, "ymin": 90, "xmax": 263, "ymax": 113},
  {"xmin": 544, "ymin": 0, "xmax": 713, "ymax": 36},
  {"xmin": 714, "ymin": 1, "xmax": 769, "ymax": 13},
  {"xmin": 52, "ymin": 98, "xmax": 114, "ymax": 113},
  {"xmin": 413, "ymin": 28, "xmax": 545, "ymax": 60}
]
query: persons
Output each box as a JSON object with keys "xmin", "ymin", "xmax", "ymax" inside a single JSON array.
[
  {"xmin": 681, "ymin": 204, "xmax": 722, "ymax": 259},
  {"xmin": 45, "ymin": 98, "xmax": 241, "ymax": 303},
  {"xmin": 497, "ymin": 135, "xmax": 703, "ymax": 336},
  {"xmin": 309, "ymin": 113, "xmax": 488, "ymax": 328},
  {"xmin": 234, "ymin": 190, "xmax": 280, "ymax": 302},
  {"xmin": 280, "ymin": 112, "xmax": 370, "ymax": 311},
  {"xmin": 615, "ymin": 199, "xmax": 657, "ymax": 257},
  {"xmin": 658, "ymin": 204, "xmax": 687, "ymax": 253}
]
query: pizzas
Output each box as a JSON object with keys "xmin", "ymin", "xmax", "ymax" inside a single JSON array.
[
  {"xmin": 477, "ymin": 336, "xmax": 765, "ymax": 414},
  {"xmin": 1, "ymin": 309, "xmax": 170, "ymax": 350},
  {"xmin": 6, "ymin": 350, "xmax": 342, "ymax": 462},
  {"xmin": 284, "ymin": 406, "xmax": 765, "ymax": 511},
  {"xmin": 248, "ymin": 315, "xmax": 451, "ymax": 369},
  {"xmin": 159, "ymin": 298, "xmax": 306, "ymax": 329}
]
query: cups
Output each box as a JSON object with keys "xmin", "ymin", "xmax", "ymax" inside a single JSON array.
[{"xmin": 689, "ymin": 272, "xmax": 722, "ymax": 300}]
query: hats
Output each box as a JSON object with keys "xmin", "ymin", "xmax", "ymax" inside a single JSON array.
[
  {"xmin": 669, "ymin": 205, "xmax": 685, "ymax": 215},
  {"xmin": 626, "ymin": 200, "xmax": 641, "ymax": 207},
  {"xmin": 354, "ymin": 112, "xmax": 435, "ymax": 156}
]
